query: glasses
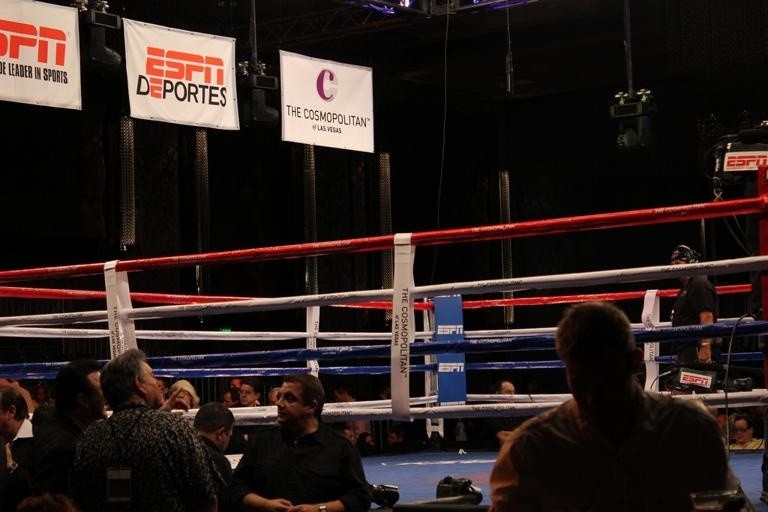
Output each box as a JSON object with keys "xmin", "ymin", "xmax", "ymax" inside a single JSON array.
[{"xmin": 734, "ymin": 427, "xmax": 748, "ymax": 433}]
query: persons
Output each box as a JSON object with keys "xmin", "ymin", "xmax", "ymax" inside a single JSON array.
[
  {"xmin": 326, "ymin": 381, "xmax": 404, "ymax": 458},
  {"xmin": 480, "ymin": 380, "xmax": 516, "ymax": 447},
  {"xmin": 0, "ymin": 348, "xmax": 371, "ymax": 511},
  {"xmin": 670, "ymin": 243, "xmax": 765, "ymax": 450},
  {"xmin": 489, "ymin": 302, "xmax": 755, "ymax": 511}
]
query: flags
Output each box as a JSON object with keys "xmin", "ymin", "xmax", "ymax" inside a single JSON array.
[
  {"xmin": 280, "ymin": 50, "xmax": 374, "ymax": 153},
  {"xmin": 0, "ymin": 1, "xmax": 84, "ymax": 111},
  {"xmin": 122, "ymin": 18, "xmax": 240, "ymax": 131}
]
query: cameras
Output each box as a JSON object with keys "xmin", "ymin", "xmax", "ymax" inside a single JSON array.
[
  {"xmin": 435, "ymin": 474, "xmax": 483, "ymax": 507},
  {"xmin": 368, "ymin": 481, "xmax": 399, "ymax": 508}
]
